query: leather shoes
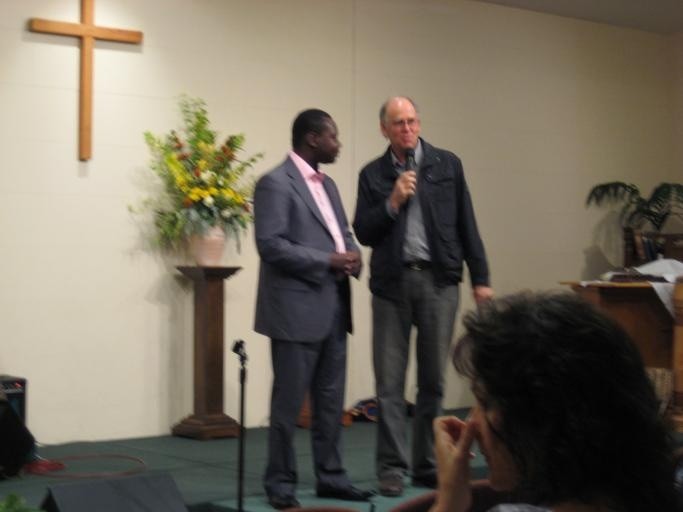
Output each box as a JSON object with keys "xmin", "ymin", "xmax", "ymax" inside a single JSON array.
[
  {"xmin": 410, "ymin": 476, "xmax": 439, "ymax": 490},
  {"xmin": 379, "ymin": 479, "xmax": 405, "ymax": 499},
  {"xmin": 315, "ymin": 484, "xmax": 375, "ymax": 501},
  {"xmin": 268, "ymin": 491, "xmax": 301, "ymax": 510}
]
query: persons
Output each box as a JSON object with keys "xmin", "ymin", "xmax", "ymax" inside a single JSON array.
[
  {"xmin": 351, "ymin": 95, "xmax": 492, "ymax": 497},
  {"xmin": 431, "ymin": 289, "xmax": 681, "ymax": 512},
  {"xmin": 254, "ymin": 108, "xmax": 377, "ymax": 508}
]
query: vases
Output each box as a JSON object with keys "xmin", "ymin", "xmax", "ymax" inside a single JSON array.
[{"xmin": 169, "ymin": 219, "xmax": 230, "ymax": 267}]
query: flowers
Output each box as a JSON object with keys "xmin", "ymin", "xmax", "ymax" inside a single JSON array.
[{"xmin": 125, "ymin": 90, "xmax": 266, "ymax": 270}]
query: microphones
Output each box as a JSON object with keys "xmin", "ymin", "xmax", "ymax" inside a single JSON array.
[{"xmin": 404, "ymin": 147, "xmax": 415, "ymax": 171}]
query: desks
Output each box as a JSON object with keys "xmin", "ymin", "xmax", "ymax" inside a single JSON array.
[{"xmin": 553, "ymin": 272, "xmax": 682, "ymax": 414}]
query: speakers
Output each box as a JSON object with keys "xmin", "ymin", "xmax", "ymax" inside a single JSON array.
[
  {"xmin": 39, "ymin": 468, "xmax": 190, "ymax": 512},
  {"xmin": 0, "ymin": 393, "xmax": 35, "ymax": 483}
]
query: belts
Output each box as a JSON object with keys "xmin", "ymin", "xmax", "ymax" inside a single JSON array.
[{"xmin": 403, "ymin": 259, "xmax": 432, "ymax": 272}]
